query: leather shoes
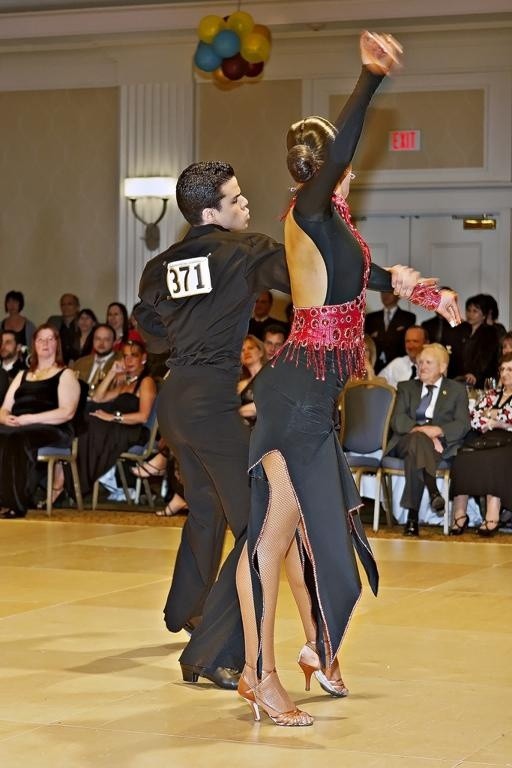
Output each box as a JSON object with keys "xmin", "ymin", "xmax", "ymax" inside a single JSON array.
[
  {"xmin": 404, "ymin": 520, "xmax": 419, "ymax": 536},
  {"xmin": 0, "ymin": 508, "xmax": 26, "ymax": 519},
  {"xmin": 430, "ymin": 493, "xmax": 445, "ymax": 517},
  {"xmin": 184, "ymin": 616, "xmax": 201, "ymax": 634},
  {"xmin": 179, "ymin": 663, "xmax": 240, "ymax": 689}
]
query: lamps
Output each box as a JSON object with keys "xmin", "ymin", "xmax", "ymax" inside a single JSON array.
[{"xmin": 124, "ymin": 177, "xmax": 178, "ymax": 251}]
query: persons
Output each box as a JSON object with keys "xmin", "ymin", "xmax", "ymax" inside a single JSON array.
[
  {"xmin": 132, "ymin": 162, "xmax": 440, "ymax": 692},
  {"xmin": 234, "ymin": 29, "xmax": 462, "ymax": 726},
  {"xmin": 1, "ymin": 289, "xmax": 512, "ymax": 536}
]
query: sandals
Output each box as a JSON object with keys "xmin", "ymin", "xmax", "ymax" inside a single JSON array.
[
  {"xmin": 37, "ymin": 489, "xmax": 65, "ymax": 510},
  {"xmin": 155, "ymin": 501, "xmax": 188, "ymax": 517},
  {"xmin": 129, "ymin": 462, "xmax": 168, "ymax": 479}
]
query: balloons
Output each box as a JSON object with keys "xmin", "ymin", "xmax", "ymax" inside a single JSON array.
[{"xmin": 189, "ymin": 12, "xmax": 274, "ymax": 91}]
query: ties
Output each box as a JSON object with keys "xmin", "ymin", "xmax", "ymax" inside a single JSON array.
[
  {"xmin": 384, "ymin": 311, "xmax": 391, "ymax": 332},
  {"xmin": 410, "ymin": 365, "xmax": 417, "ymax": 379},
  {"xmin": 415, "ymin": 385, "xmax": 434, "ymax": 426},
  {"xmin": 91, "ymin": 361, "xmax": 103, "ymax": 384}
]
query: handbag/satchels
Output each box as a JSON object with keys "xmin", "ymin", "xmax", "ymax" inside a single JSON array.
[{"xmin": 465, "ymin": 427, "xmax": 510, "ymax": 448}]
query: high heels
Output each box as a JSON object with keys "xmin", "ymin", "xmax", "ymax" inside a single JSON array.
[
  {"xmin": 448, "ymin": 514, "xmax": 469, "ymax": 536},
  {"xmin": 238, "ymin": 661, "xmax": 314, "ymax": 726},
  {"xmin": 476, "ymin": 520, "xmax": 501, "ymax": 537},
  {"xmin": 297, "ymin": 640, "xmax": 349, "ymax": 698}
]
